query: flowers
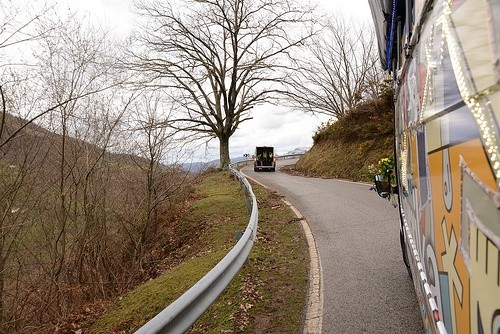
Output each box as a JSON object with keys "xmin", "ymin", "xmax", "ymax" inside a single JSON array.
[{"xmin": 367, "ymin": 157, "xmax": 394, "ymax": 179}]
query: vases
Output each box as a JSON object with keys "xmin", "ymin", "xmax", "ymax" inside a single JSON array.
[{"xmin": 373, "ymin": 172, "xmax": 393, "ymax": 197}]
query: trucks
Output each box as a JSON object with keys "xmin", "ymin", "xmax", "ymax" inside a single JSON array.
[{"xmin": 252, "ymin": 146, "xmax": 276, "ymax": 172}]
